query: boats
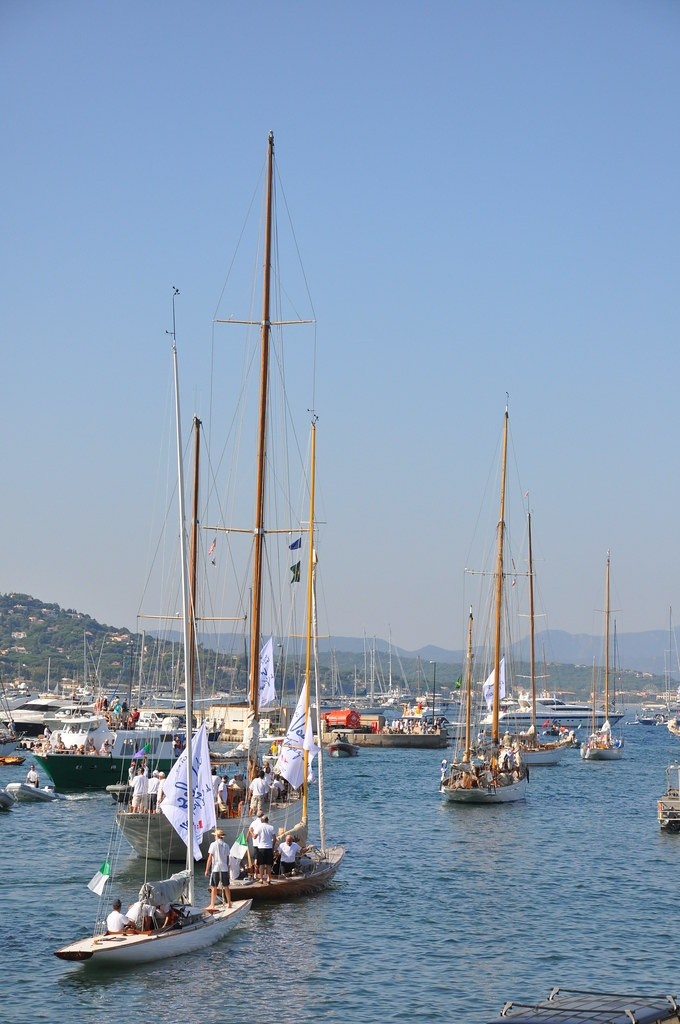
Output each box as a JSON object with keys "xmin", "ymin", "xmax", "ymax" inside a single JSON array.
[
  {"xmin": 326, "ymin": 728, "xmax": 362, "ymax": 759},
  {"xmin": 5, "ymin": 782, "xmax": 66, "ymax": 803},
  {"xmin": 0, "ymin": 755, "xmax": 26, "ymax": 766},
  {"xmin": 657, "ymin": 759, "xmax": 680, "ymax": 832},
  {"xmin": 0, "ymin": 788, "xmax": 15, "ymax": 810}
]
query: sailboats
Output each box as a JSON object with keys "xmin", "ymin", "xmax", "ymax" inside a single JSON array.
[
  {"xmin": 53, "ymin": 284, "xmax": 256, "ymax": 971},
  {"xmin": 438, "ymin": 391, "xmax": 543, "ymax": 805},
  {"xmin": 1, "ymin": 123, "xmax": 677, "ymax": 903},
  {"xmin": 578, "ymin": 549, "xmax": 626, "ymax": 761}
]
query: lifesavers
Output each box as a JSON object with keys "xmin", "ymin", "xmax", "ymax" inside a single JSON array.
[{"xmin": 103, "ymin": 713, "xmax": 112, "ymax": 726}]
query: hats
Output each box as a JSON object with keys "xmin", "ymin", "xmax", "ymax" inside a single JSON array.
[
  {"xmin": 137, "ymin": 767, "xmax": 144, "ymax": 771},
  {"xmin": 113, "ymin": 899, "xmax": 121, "ymax": 907},
  {"xmin": 131, "ymin": 761, "xmax": 136, "ymax": 766},
  {"xmin": 158, "ymin": 772, "xmax": 165, "ymax": 777},
  {"xmin": 211, "ymin": 829, "xmax": 225, "ymax": 837},
  {"xmin": 152, "ymin": 770, "xmax": 159, "ymax": 776}
]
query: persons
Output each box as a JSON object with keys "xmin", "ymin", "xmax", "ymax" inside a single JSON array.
[
  {"xmin": 106, "ymin": 899, "xmax": 136, "ymax": 935},
  {"xmin": 125, "ymin": 901, "xmax": 156, "ymax": 931},
  {"xmin": 205, "ymin": 830, "xmax": 232, "ymax": 909},
  {"xmin": 382, "ymin": 702, "xmax": 523, "ymax": 789},
  {"xmin": 249, "ymin": 811, "xmax": 276, "ymax": 884},
  {"xmin": 274, "ymin": 835, "xmax": 314, "ymax": 877},
  {"xmin": 524, "ymin": 720, "xmax": 620, "ymax": 748},
  {"xmin": 229, "ymin": 856, "xmax": 248, "ymax": 880},
  {"xmin": 7, "ymin": 691, "xmax": 140, "ymax": 755},
  {"xmin": 211, "ymin": 762, "xmax": 289, "ymax": 817},
  {"xmin": 129, "ymin": 762, "xmax": 166, "ymax": 815},
  {"xmin": 155, "ymin": 905, "xmax": 171, "ymax": 928},
  {"xmin": 335, "ymin": 733, "xmax": 348, "ymax": 742},
  {"xmin": 26, "ymin": 765, "xmax": 39, "ymax": 788}
]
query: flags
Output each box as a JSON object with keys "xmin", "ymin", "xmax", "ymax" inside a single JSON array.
[
  {"xmin": 160, "ymin": 725, "xmax": 216, "ymax": 862},
  {"xmin": 135, "ymin": 742, "xmax": 151, "ymax": 757},
  {"xmin": 288, "ymin": 538, "xmax": 302, "ymax": 583},
  {"xmin": 454, "ymin": 675, "xmax": 462, "ymax": 688},
  {"xmin": 482, "ymin": 658, "xmax": 506, "ymax": 710},
  {"xmin": 209, "ymin": 538, "xmax": 216, "ymax": 567},
  {"xmin": 273, "ymin": 681, "xmax": 320, "ymax": 791},
  {"xmin": 87, "ymin": 858, "xmax": 111, "ymax": 896},
  {"xmin": 230, "ymin": 831, "xmax": 248, "ymax": 859},
  {"xmin": 542, "ymin": 718, "xmax": 550, "ymax": 729},
  {"xmin": 248, "ymin": 637, "xmax": 276, "ymax": 709}
]
